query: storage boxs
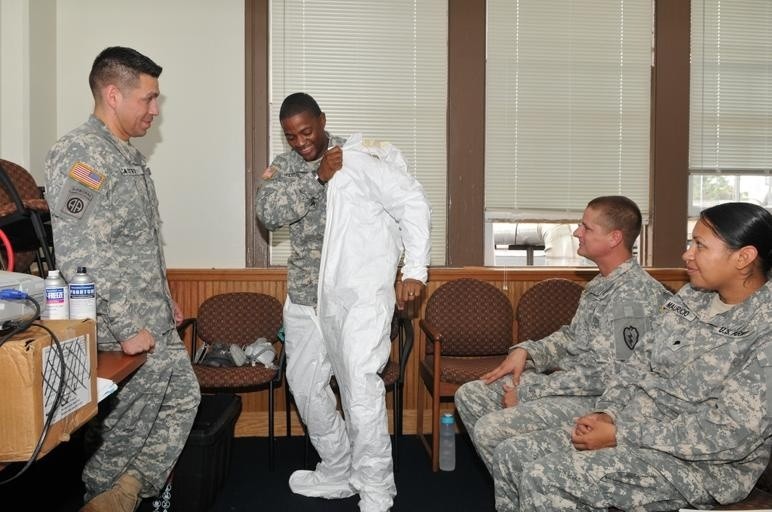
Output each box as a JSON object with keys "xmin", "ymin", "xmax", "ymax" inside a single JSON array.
[
  {"xmin": 172, "ymin": 391, "xmax": 243, "ymax": 512},
  {"xmin": 0, "ymin": 317, "xmax": 100, "ymax": 465}
]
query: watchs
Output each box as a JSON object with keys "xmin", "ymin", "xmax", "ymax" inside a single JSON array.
[{"xmin": 312, "ymin": 170, "xmax": 324, "ymax": 186}]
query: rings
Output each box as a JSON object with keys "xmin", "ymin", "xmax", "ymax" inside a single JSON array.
[{"xmin": 408, "ymin": 293, "xmax": 415, "ymax": 295}]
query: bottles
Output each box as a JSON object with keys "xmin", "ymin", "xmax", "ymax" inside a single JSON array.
[
  {"xmin": 42, "ymin": 266, "xmax": 96, "ymax": 320},
  {"xmin": 439, "ymin": 413, "xmax": 456, "ymax": 471}
]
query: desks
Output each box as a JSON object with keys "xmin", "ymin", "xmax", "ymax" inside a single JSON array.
[{"xmin": 0, "ymin": 349, "xmax": 148, "ymax": 472}]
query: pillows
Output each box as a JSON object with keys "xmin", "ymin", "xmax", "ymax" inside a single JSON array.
[{"xmin": 515, "ymin": 276, "xmax": 585, "ymax": 375}]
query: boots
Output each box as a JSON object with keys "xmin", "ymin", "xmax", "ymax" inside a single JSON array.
[{"xmin": 79, "ymin": 473, "xmax": 144, "ymax": 511}]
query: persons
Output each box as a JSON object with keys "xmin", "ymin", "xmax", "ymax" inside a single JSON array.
[
  {"xmin": 494, "ymin": 202, "xmax": 771, "ymax": 512},
  {"xmin": 43, "ymin": 46, "xmax": 201, "ymax": 512},
  {"xmin": 253, "ymin": 93, "xmax": 432, "ymax": 512},
  {"xmin": 454, "ymin": 196, "xmax": 674, "ymax": 478}
]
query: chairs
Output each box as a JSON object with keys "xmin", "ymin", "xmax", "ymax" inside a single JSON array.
[
  {"xmin": 0, "ymin": 157, "xmax": 55, "ymax": 281},
  {"xmin": 710, "ymin": 456, "xmax": 772, "ymax": 510},
  {"xmin": 284, "ymin": 304, "xmax": 415, "ymax": 471},
  {"xmin": 429, "ymin": 281, "xmax": 512, "ymax": 466},
  {"xmin": 174, "ymin": 291, "xmax": 294, "ymax": 474}
]
